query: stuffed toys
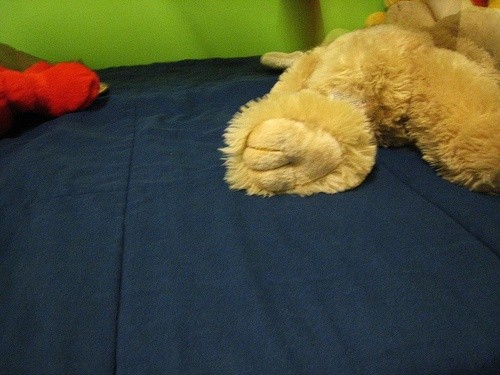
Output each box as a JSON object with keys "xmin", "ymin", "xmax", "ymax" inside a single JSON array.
[
  {"xmin": 217, "ymin": 0, "xmax": 500, "ymax": 199},
  {"xmin": 0, "ymin": 60, "xmax": 99, "ymax": 120}
]
